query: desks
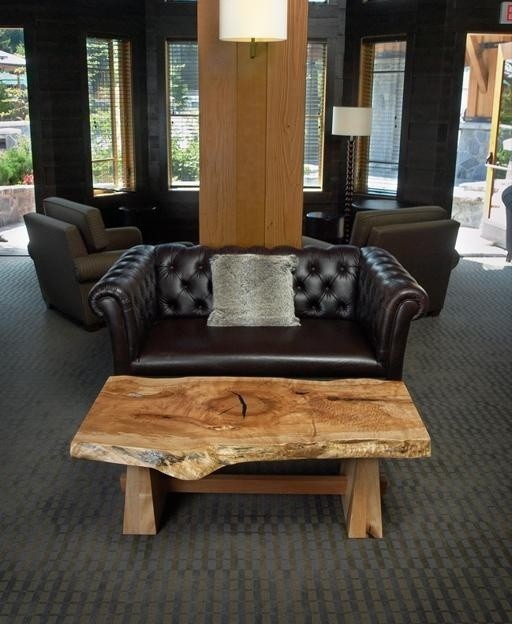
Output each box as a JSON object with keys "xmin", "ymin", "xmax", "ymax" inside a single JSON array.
[
  {"xmin": 70, "ymin": 373, "xmax": 433, "ymax": 539},
  {"xmin": 351, "ymin": 198, "xmax": 419, "ymax": 211},
  {"xmin": 117, "ymin": 203, "xmax": 161, "ymax": 230}
]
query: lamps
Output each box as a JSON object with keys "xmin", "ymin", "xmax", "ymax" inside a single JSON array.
[
  {"xmin": 219, "ymin": 0, "xmax": 288, "ymax": 58},
  {"xmin": 331, "ymin": 105, "xmax": 374, "ymax": 249}
]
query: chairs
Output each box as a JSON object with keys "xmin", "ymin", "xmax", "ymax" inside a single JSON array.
[
  {"xmin": 23, "ymin": 212, "xmax": 143, "ymax": 332},
  {"xmin": 349, "ymin": 205, "xmax": 460, "ymax": 315}
]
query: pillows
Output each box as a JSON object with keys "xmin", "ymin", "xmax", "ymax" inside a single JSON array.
[{"xmin": 206, "ymin": 253, "xmax": 301, "ymax": 328}]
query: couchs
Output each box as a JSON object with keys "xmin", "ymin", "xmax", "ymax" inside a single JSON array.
[{"xmin": 87, "ymin": 243, "xmax": 429, "ymax": 380}]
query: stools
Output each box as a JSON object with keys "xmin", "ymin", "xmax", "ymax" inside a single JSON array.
[{"xmin": 305, "ymin": 212, "xmax": 345, "ymax": 243}]
